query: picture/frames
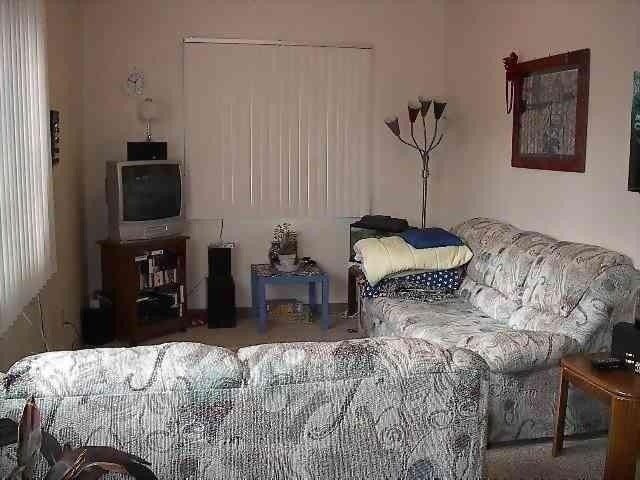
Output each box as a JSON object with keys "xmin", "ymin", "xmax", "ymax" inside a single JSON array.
[{"xmin": 511, "ymin": 49, "xmax": 591, "ymax": 173}]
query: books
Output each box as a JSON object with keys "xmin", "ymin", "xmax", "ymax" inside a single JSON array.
[{"xmin": 128, "ymin": 247, "xmax": 185, "ymax": 321}]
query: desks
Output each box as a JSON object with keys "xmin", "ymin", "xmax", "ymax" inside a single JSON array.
[{"xmin": 251, "ymin": 263, "xmax": 329, "ymax": 335}]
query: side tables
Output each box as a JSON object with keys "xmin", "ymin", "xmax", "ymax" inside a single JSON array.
[{"xmin": 551, "ymin": 351, "xmax": 640, "ymax": 480}]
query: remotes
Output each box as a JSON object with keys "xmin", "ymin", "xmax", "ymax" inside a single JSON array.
[{"xmin": 591, "ymin": 355, "xmax": 625, "ymax": 369}]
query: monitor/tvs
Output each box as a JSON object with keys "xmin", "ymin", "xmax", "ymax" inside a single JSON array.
[{"xmin": 105, "ymin": 160, "xmax": 186, "ymax": 245}]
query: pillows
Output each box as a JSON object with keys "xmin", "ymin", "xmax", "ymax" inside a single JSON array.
[{"xmin": 399, "ymin": 227, "xmax": 464, "ymax": 249}]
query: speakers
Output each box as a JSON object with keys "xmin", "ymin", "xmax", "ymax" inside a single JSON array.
[
  {"xmin": 207, "ymin": 245, "xmax": 232, "ymax": 281},
  {"xmin": 80, "ymin": 303, "xmax": 115, "ymax": 348},
  {"xmin": 206, "ymin": 277, "xmax": 236, "ymax": 329}
]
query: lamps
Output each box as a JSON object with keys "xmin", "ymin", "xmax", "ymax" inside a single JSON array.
[
  {"xmin": 142, "ymin": 97, "xmax": 153, "ymax": 139},
  {"xmin": 383, "ymin": 95, "xmax": 447, "ymax": 230}
]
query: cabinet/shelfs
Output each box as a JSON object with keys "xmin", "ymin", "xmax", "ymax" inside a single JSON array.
[{"xmin": 96, "ymin": 235, "xmax": 189, "ymax": 345}]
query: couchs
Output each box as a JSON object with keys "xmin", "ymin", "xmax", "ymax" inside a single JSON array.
[
  {"xmin": 353, "ymin": 217, "xmax": 640, "ymax": 447},
  {"xmin": 0, "ymin": 336, "xmax": 490, "ymax": 480}
]
